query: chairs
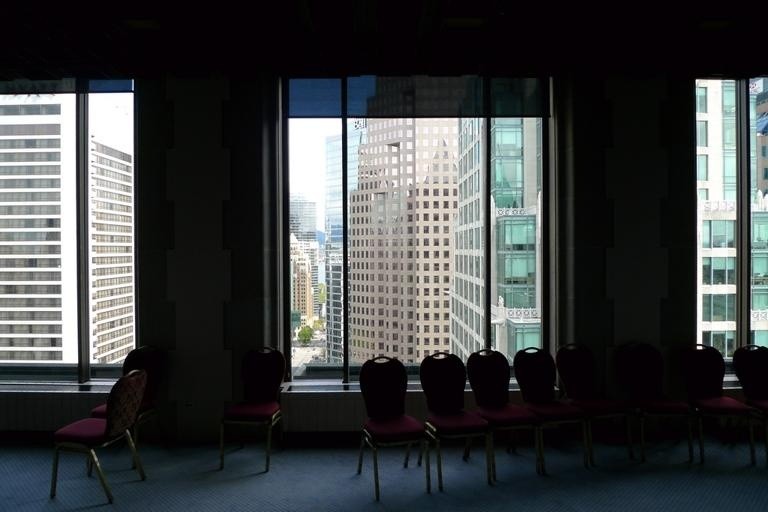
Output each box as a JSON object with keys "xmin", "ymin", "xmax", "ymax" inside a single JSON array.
[
  {"xmin": 463, "ymin": 348, "xmax": 545, "ymax": 479},
  {"xmin": 50, "ymin": 344, "xmax": 163, "ymax": 506},
  {"xmin": 218, "ymin": 343, "xmax": 287, "ymax": 474},
  {"xmin": 357, "ymin": 355, "xmax": 431, "ymax": 501},
  {"xmin": 418, "ymin": 351, "xmax": 493, "ymax": 490},
  {"xmin": 512, "ymin": 347, "xmax": 592, "ymax": 475},
  {"xmin": 556, "ymin": 341, "xmax": 768, "ymax": 470}
]
